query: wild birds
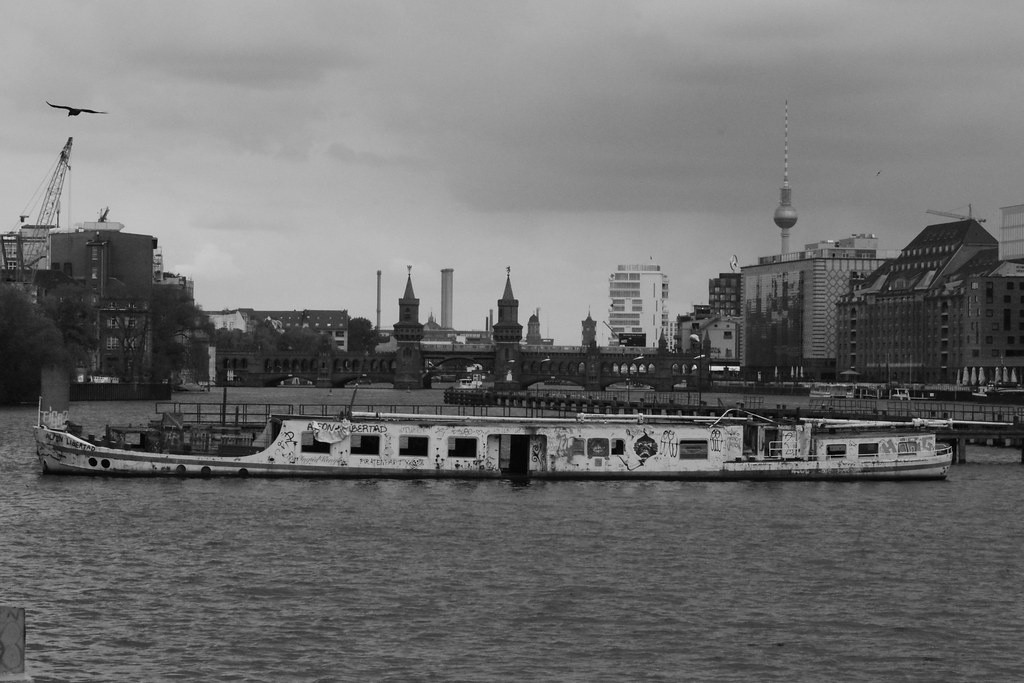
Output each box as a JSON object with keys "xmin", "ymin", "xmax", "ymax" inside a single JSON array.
[{"xmin": 45, "ymin": 100, "xmax": 108, "ymax": 117}]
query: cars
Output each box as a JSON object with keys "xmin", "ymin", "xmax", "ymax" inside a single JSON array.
[
  {"xmin": 423, "ymin": 359, "xmax": 494, "ymax": 390},
  {"xmin": 802, "ymin": 371, "xmax": 1023, "ymax": 406}
]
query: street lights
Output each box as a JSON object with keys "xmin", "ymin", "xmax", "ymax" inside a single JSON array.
[
  {"xmin": 689, "ymin": 333, "xmax": 702, "ymax": 413},
  {"xmin": 538, "ymin": 358, "xmax": 551, "ymax": 418},
  {"xmin": 507, "ymin": 359, "xmax": 516, "ymax": 417}
]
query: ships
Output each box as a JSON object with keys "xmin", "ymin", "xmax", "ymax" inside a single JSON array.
[{"xmin": 29, "ymin": 376, "xmax": 954, "ymax": 482}]
